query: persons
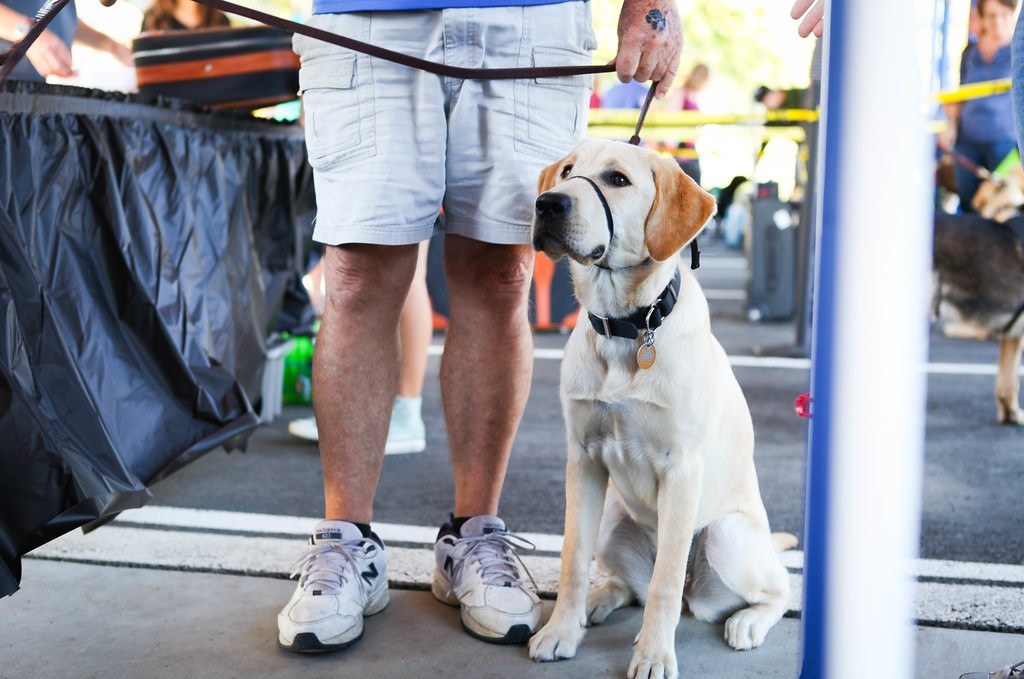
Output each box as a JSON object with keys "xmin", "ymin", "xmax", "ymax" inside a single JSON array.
[
  {"xmin": 139, "ymin": 0, "xmax": 232, "ymax": 35},
  {"xmin": 278, "ymin": 0, "xmax": 684, "ymax": 654},
  {"xmin": 0, "ymin": 1, "xmax": 135, "ymax": 87},
  {"xmin": 292, "ymin": 217, "xmax": 434, "ymax": 456},
  {"xmin": 591, "ymin": 1, "xmax": 1024, "ymax": 304}
]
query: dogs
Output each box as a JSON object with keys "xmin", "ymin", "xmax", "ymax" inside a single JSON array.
[
  {"xmin": 926, "ymin": 206, "xmax": 1024, "ymax": 426},
  {"xmin": 526, "ymin": 139, "xmax": 800, "ymax": 679}
]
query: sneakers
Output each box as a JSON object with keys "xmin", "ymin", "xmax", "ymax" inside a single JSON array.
[
  {"xmin": 288, "ymin": 393, "xmax": 427, "ymax": 456},
  {"xmin": 277, "ymin": 520, "xmax": 391, "ymax": 654},
  {"xmin": 430, "ymin": 512, "xmax": 544, "ymax": 644}
]
